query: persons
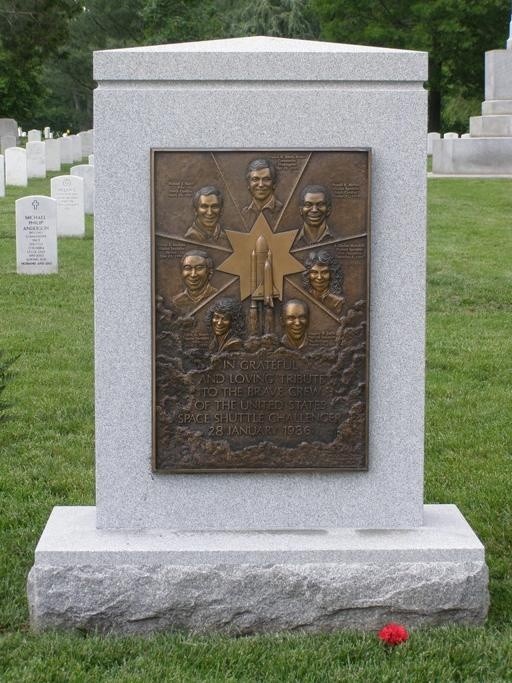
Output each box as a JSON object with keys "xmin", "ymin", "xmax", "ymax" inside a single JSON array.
[
  {"xmin": 183, "ymin": 185, "xmax": 228, "ymax": 248},
  {"xmin": 277, "ymin": 297, "xmax": 320, "ymax": 354},
  {"xmin": 171, "ymin": 248, "xmax": 219, "ymax": 303},
  {"xmin": 293, "ymin": 183, "xmax": 335, "ymax": 246},
  {"xmin": 301, "ymin": 248, "xmax": 345, "ymax": 312},
  {"xmin": 206, "ymin": 295, "xmax": 250, "ymax": 356},
  {"xmin": 240, "ymin": 157, "xmax": 284, "ymax": 218}
]
